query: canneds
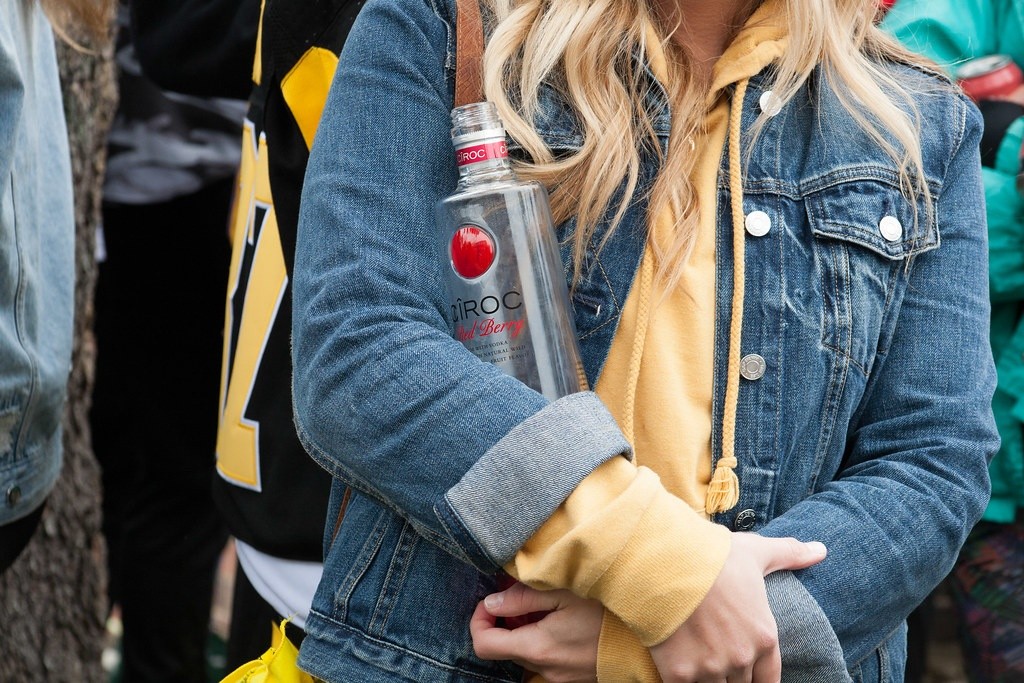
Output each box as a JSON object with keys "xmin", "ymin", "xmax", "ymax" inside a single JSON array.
[{"xmin": 955, "ymin": 53, "xmax": 1024, "ymax": 105}]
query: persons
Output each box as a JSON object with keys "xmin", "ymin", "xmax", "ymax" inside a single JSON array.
[
  {"xmin": 878, "ymin": 0, "xmax": 1024, "ymax": 683},
  {"xmin": 290, "ymin": 0, "xmax": 999, "ymax": 683},
  {"xmin": 0, "ymin": 2, "xmax": 80, "ymax": 583},
  {"xmin": 227, "ymin": 0, "xmax": 389, "ymax": 675}
]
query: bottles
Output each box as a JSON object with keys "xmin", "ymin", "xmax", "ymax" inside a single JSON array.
[{"xmin": 434, "ymin": 102, "xmax": 580, "ymax": 398}]
